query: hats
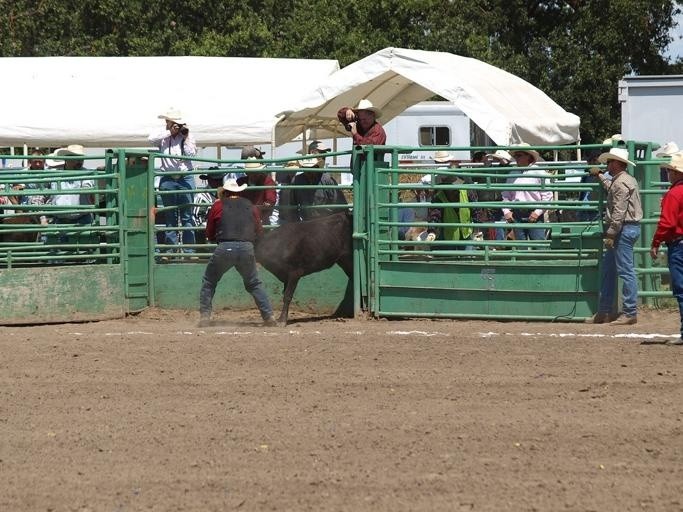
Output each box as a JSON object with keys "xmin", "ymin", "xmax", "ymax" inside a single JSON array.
[
  {"xmin": 429, "ymin": 151, "xmax": 454, "ymax": 162},
  {"xmin": 57, "ymin": 145, "xmax": 86, "ymax": 157},
  {"xmin": 508, "ymin": 143, "xmax": 539, "ymax": 159},
  {"xmin": 158, "ymin": 107, "xmax": 187, "ymax": 125},
  {"xmin": 199, "ymin": 147, "xmax": 267, "ymax": 193},
  {"xmin": 308, "ymin": 140, "xmax": 331, "ymax": 152},
  {"xmin": 660, "ymin": 150, "xmax": 683, "ymax": 172},
  {"xmin": 352, "ymin": 99, "xmax": 383, "ymax": 118},
  {"xmin": 46, "ymin": 148, "xmax": 66, "ymax": 166},
  {"xmin": 486, "ymin": 150, "xmax": 512, "ymax": 161},
  {"xmin": 598, "ymin": 148, "xmax": 636, "ymax": 166},
  {"xmin": 299, "ymin": 155, "xmax": 324, "ymax": 168}
]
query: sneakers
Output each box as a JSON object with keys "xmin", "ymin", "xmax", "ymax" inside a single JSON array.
[
  {"xmin": 198, "ymin": 318, "xmax": 210, "ymax": 328},
  {"xmin": 585, "ymin": 313, "xmax": 617, "ymax": 323},
  {"xmin": 610, "ymin": 315, "xmax": 636, "ymax": 325},
  {"xmin": 258, "ymin": 316, "xmax": 277, "ymax": 328},
  {"xmin": 670, "ymin": 339, "xmax": 683, "ymax": 345}
]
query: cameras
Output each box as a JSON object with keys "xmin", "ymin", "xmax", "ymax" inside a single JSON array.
[
  {"xmin": 344, "ymin": 118, "xmax": 360, "ymax": 132},
  {"xmin": 176, "ymin": 125, "xmax": 187, "ymax": 135}
]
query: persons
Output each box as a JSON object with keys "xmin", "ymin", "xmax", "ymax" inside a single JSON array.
[
  {"xmin": 148, "ymin": 110, "xmax": 199, "ymax": 260},
  {"xmin": 193, "ymin": 146, "xmax": 280, "ymax": 260},
  {"xmin": 583, "ymin": 145, "xmax": 644, "ymax": 327},
  {"xmin": 414, "ymin": 143, "xmax": 553, "ymax": 258},
  {"xmin": 337, "ymin": 99, "xmax": 386, "ymax": 169},
  {"xmin": 275, "ymin": 139, "xmax": 349, "ymax": 223},
  {"xmin": 195, "ymin": 178, "xmax": 280, "ymax": 329},
  {"xmin": 647, "ymin": 153, "xmax": 682, "ymax": 347},
  {"xmin": 7, "ymin": 145, "xmax": 94, "ymax": 264}
]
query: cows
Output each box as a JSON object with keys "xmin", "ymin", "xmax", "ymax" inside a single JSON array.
[{"xmin": 254, "ymin": 211, "xmax": 353, "ymax": 327}]
